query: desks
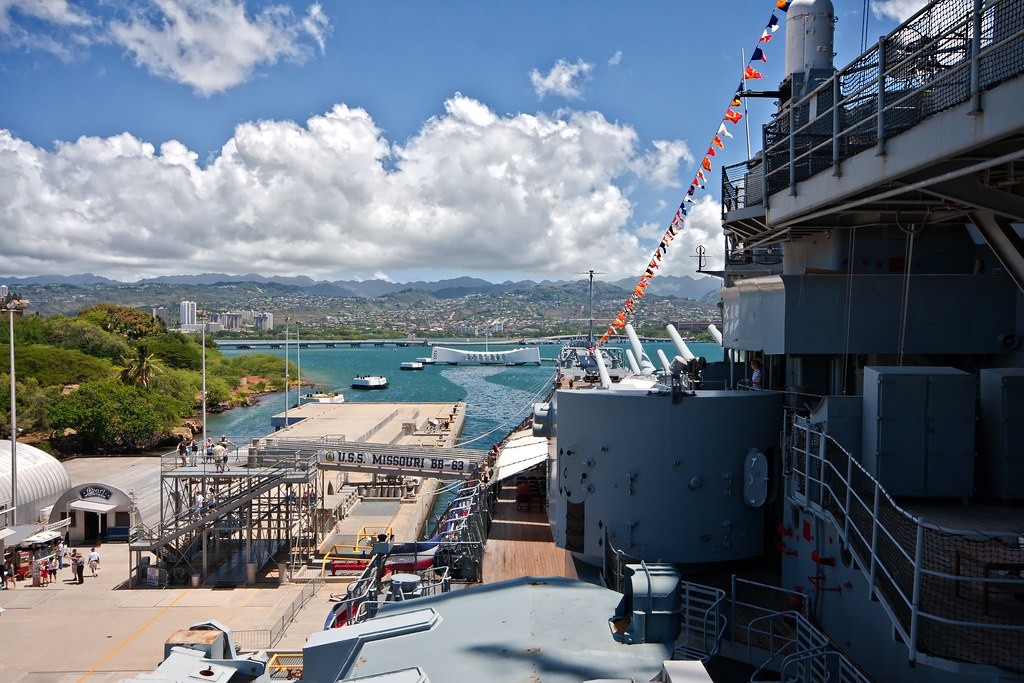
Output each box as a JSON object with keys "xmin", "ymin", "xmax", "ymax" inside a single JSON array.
[{"xmin": 954, "ymin": 544, "xmax": 1024, "ymax": 615}]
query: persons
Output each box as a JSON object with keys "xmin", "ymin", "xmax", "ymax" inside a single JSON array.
[
  {"xmin": 492, "ymin": 444, "xmax": 499, "ymax": 453},
  {"xmin": 176, "ymin": 434, "xmax": 235, "ymax": 473},
  {"xmin": 87, "ymin": 547, "xmax": 99, "ymax": 577},
  {"xmin": 284, "ymin": 489, "xmax": 315, "ymax": 506},
  {"xmin": 194, "ymin": 488, "xmax": 223, "ymax": 519},
  {"xmin": 0, "ymin": 540, "xmax": 64, "ymax": 590},
  {"xmin": 569, "ymin": 379, "xmax": 573, "ymax": 389},
  {"xmin": 516, "ymin": 480, "xmax": 531, "ymax": 496},
  {"xmin": 70, "ymin": 549, "xmax": 84, "ymax": 585},
  {"xmin": 427, "ymin": 418, "xmax": 432, "ymax": 431},
  {"xmin": 747, "ymin": 359, "xmax": 763, "ymax": 392}
]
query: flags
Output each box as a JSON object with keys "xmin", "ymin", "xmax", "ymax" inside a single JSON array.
[{"xmin": 589, "ymin": 0, "xmax": 791, "ymax": 355}]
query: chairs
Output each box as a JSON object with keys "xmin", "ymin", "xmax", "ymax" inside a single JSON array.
[{"xmin": 516, "ymin": 476, "xmax": 546, "ymax": 512}]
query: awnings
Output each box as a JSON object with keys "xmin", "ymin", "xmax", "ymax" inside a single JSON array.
[{"xmin": 486, "ymin": 429, "xmax": 549, "ymax": 487}]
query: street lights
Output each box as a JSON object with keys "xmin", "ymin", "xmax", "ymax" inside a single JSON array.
[
  {"xmin": 284, "ymin": 317, "xmax": 292, "ymax": 429},
  {"xmin": 195, "ymin": 309, "xmax": 213, "ymax": 450},
  {"xmin": 0, "ymin": 300, "xmax": 30, "ymax": 526},
  {"xmin": 295, "ymin": 320, "xmax": 303, "ymax": 408}
]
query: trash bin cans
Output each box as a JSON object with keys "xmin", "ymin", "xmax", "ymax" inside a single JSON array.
[
  {"xmin": 401, "ymin": 485, "xmax": 407, "ymax": 497},
  {"xmin": 141, "ymin": 556, "xmax": 151, "ymax": 578},
  {"xmin": 395, "ymin": 485, "xmax": 401, "ymax": 497},
  {"xmin": 414, "ymin": 485, "xmax": 419, "ymax": 494},
  {"xmin": 382, "ymin": 485, "xmax": 388, "ymax": 497},
  {"xmin": 367, "ymin": 485, "xmax": 373, "ymax": 497},
  {"xmin": 389, "ymin": 485, "xmax": 394, "ymax": 497},
  {"xmin": 278, "ymin": 563, "xmax": 286, "ymax": 584},
  {"xmin": 359, "ymin": 485, "xmax": 366, "ymax": 496},
  {"xmin": 376, "ymin": 485, "xmax": 381, "ymax": 497},
  {"xmin": 191, "ymin": 574, "xmax": 200, "ymax": 588},
  {"xmin": 246, "ymin": 564, "xmax": 256, "ymax": 586}
]
